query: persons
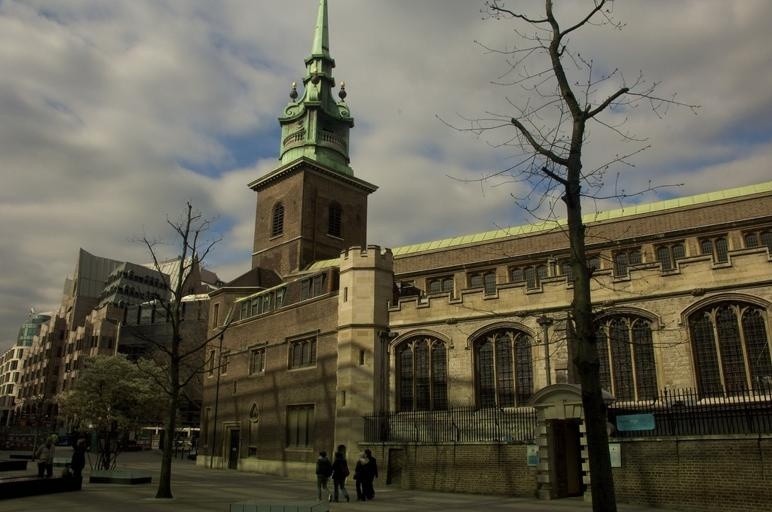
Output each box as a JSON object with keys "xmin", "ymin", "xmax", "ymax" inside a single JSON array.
[
  {"xmin": 71, "ymin": 432, "xmax": 92, "ymax": 476},
  {"xmin": 332, "ymin": 451, "xmax": 350, "ymax": 502},
  {"xmin": 190, "ymin": 431, "xmax": 197, "ymax": 448},
  {"xmin": 316, "ymin": 450, "xmax": 333, "ymax": 502},
  {"xmin": 365, "ymin": 448, "xmax": 380, "ymax": 502},
  {"xmin": 35, "ymin": 435, "xmax": 57, "ymax": 476},
  {"xmin": 353, "ymin": 451, "xmax": 372, "ymax": 503}
]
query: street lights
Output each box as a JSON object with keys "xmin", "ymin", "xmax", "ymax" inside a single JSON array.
[
  {"xmin": 378, "ymin": 328, "xmax": 398, "ymax": 414},
  {"xmin": 536, "ymin": 315, "xmax": 554, "ymax": 387}
]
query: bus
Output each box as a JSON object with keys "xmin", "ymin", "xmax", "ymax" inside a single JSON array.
[{"xmin": 137, "ymin": 427, "xmax": 200, "ymax": 451}]
